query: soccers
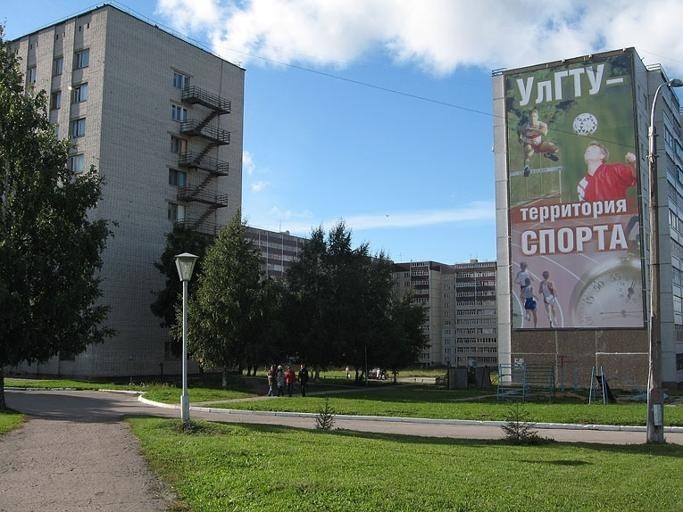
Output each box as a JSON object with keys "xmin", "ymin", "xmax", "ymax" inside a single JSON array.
[{"xmin": 573, "ymin": 113, "xmax": 598, "ymax": 136}]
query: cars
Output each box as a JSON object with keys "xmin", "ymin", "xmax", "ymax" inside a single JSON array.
[{"xmin": 368, "ymin": 367, "xmax": 385, "ymax": 380}]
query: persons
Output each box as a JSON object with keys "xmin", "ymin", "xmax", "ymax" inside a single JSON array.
[
  {"xmin": 520, "ymin": 278, "xmax": 539, "ymax": 328},
  {"xmin": 297, "ymin": 363, "xmax": 308, "ymax": 398},
  {"xmin": 519, "ymin": 108, "xmax": 560, "ymax": 176},
  {"xmin": 267, "ymin": 365, "xmax": 277, "ymax": 396},
  {"xmin": 578, "ymin": 139, "xmax": 638, "ymax": 210},
  {"xmin": 284, "ymin": 365, "xmax": 296, "ymax": 396},
  {"xmin": 506, "ymin": 75, "xmax": 521, "ymax": 118},
  {"xmin": 514, "ymin": 262, "xmax": 542, "ymax": 306},
  {"xmin": 539, "ymin": 270, "xmax": 559, "ymax": 328},
  {"xmin": 345, "ymin": 365, "xmax": 350, "ymax": 379},
  {"xmin": 276, "ymin": 365, "xmax": 285, "ymax": 397}
]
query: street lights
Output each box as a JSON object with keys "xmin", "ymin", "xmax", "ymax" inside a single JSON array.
[
  {"xmin": 174, "ymin": 252, "xmax": 200, "ymax": 424},
  {"xmin": 645, "ymin": 78, "xmax": 682, "ymax": 444}
]
